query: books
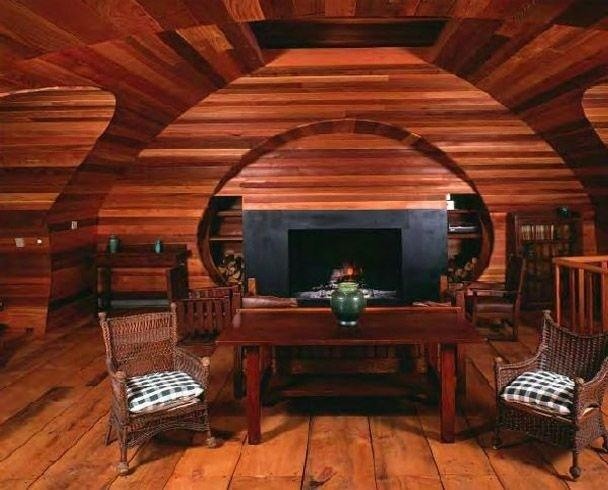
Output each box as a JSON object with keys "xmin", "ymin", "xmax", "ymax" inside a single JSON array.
[{"xmin": 521, "ymin": 224, "xmax": 570, "ymax": 257}]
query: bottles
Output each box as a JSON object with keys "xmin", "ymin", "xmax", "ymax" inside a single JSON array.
[
  {"xmin": 107, "ymin": 234, "xmax": 120, "ymax": 253},
  {"xmin": 153, "ymin": 239, "xmax": 161, "ymax": 253},
  {"xmin": 446, "ymin": 193, "xmax": 454, "ymax": 211}
]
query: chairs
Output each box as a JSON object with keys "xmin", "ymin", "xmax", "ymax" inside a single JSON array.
[
  {"xmin": 491, "ymin": 309, "xmax": 608, "ymax": 481},
  {"xmin": 175, "ymin": 285, "xmax": 240, "ymax": 398},
  {"xmin": 98, "ymin": 302, "xmax": 218, "ymax": 475},
  {"xmin": 463, "ymin": 254, "xmax": 526, "ymax": 342}
]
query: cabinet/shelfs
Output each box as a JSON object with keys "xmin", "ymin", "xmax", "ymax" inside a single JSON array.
[
  {"xmin": 509, "ymin": 210, "xmax": 580, "ymax": 310},
  {"xmin": 197, "ymin": 195, "xmax": 243, "ymax": 293},
  {"xmin": 447, "ymin": 193, "xmax": 494, "ymax": 292}
]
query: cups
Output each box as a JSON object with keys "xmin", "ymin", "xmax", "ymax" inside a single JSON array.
[{"xmin": 14, "ymin": 238, "xmax": 26, "ymax": 247}]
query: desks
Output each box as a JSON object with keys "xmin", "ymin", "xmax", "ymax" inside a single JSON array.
[
  {"xmin": 215, "ymin": 310, "xmax": 483, "ymax": 444},
  {"xmin": 81, "ymin": 244, "xmax": 192, "ymax": 316}
]
